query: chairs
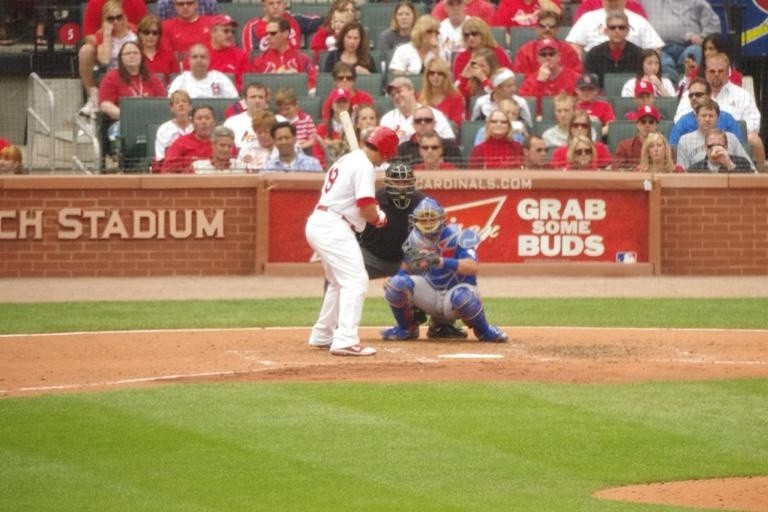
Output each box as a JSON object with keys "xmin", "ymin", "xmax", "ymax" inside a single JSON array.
[
  {"xmin": 487, "ymin": 27, "xmax": 506, "ymax": 54},
  {"xmin": 383, "ymin": 74, "xmax": 432, "ymax": 99},
  {"xmin": 603, "ymin": 73, "xmax": 672, "ymax": 100},
  {"xmin": 374, "ymin": 96, "xmax": 395, "ymax": 118},
  {"xmin": 611, "ymin": 96, "xmax": 678, "ymax": 123},
  {"xmin": 169, "ymin": 74, "xmax": 235, "ymax": 91},
  {"xmin": 219, "ymin": 3, "xmax": 262, "ymax": 48},
  {"xmin": 513, "ymin": 74, "xmax": 526, "ymax": 91},
  {"xmin": 461, "ymin": 121, "xmax": 532, "ymax": 164},
  {"xmin": 249, "ymin": 48, "xmax": 314, "ymax": 67},
  {"xmin": 96, "ymin": 72, "xmax": 166, "ymax": 91},
  {"xmin": 736, "ymin": 120, "xmax": 748, "ymax": 148},
  {"xmin": 607, "ymin": 119, "xmax": 673, "ymax": 155},
  {"xmin": 315, "ymin": 71, "xmax": 383, "ymax": 98},
  {"xmin": 288, "ymin": 3, "xmax": 307, "ymax": 47},
  {"xmin": 364, "ymin": 3, "xmax": 430, "ymax": 46},
  {"xmin": 119, "ymin": 96, "xmax": 176, "ymax": 173},
  {"xmin": 269, "ymin": 99, "xmax": 319, "ymax": 124},
  {"xmin": 190, "ymin": 98, "xmax": 238, "ymax": 127},
  {"xmin": 451, "ymin": 52, "xmax": 462, "ymax": 72},
  {"xmin": 471, "ymin": 95, "xmax": 537, "ymax": 124},
  {"xmin": 307, "ymin": 3, "xmax": 337, "ymax": 46},
  {"xmin": 542, "ymin": 96, "xmax": 611, "ymax": 123},
  {"xmin": 535, "ymin": 120, "xmax": 602, "ymax": 147},
  {"xmin": 146, "ymin": 122, "xmax": 164, "ymax": 165},
  {"xmin": 319, "ymin": 50, "xmax": 383, "ymax": 73},
  {"xmin": 510, "ymin": 27, "xmax": 572, "ymax": 63},
  {"xmin": 242, "ymin": 73, "xmax": 308, "ymax": 99},
  {"xmin": 384, "ymin": 50, "xmax": 395, "ymax": 70}
]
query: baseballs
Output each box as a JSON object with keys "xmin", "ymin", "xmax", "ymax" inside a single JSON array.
[{"xmin": 420, "ymin": 261, "xmax": 429, "ymax": 271}]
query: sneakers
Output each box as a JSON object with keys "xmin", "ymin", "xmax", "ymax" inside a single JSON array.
[
  {"xmin": 473, "ymin": 326, "xmax": 509, "ymax": 342},
  {"xmin": 306, "ymin": 340, "xmax": 332, "ymax": 350},
  {"xmin": 76, "ymin": 94, "xmax": 99, "ymax": 118},
  {"xmin": 427, "ymin": 322, "xmax": 468, "ymax": 339},
  {"xmin": 329, "ymin": 342, "xmax": 378, "ymax": 357},
  {"xmin": 382, "ymin": 325, "xmax": 421, "ymax": 342}
]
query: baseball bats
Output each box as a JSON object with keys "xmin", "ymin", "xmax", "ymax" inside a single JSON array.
[{"xmin": 339, "ymin": 110, "xmax": 379, "ymax": 211}]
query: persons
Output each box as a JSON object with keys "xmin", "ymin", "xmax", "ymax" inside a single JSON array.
[
  {"xmin": 473, "ymin": 95, "xmax": 530, "ymax": 145},
  {"xmin": 415, "ymin": 56, "xmax": 467, "ymax": 128},
  {"xmin": 161, "ymin": 0, "xmax": 215, "ymax": 73},
  {"xmin": 235, "ymin": 109, "xmax": 279, "ymax": 171},
  {"xmin": 0, "ymin": 133, "xmax": 10, "ymax": 152},
  {"xmin": 541, "ymin": 87, "xmax": 599, "ymax": 145},
  {"xmin": 303, "ymin": 124, "xmax": 402, "ymax": 358},
  {"xmin": 387, "ymin": 13, "xmax": 449, "ymax": 76},
  {"xmin": 376, "ymin": 0, "xmax": 417, "ymax": 76},
  {"xmin": 623, "ymin": 79, "xmax": 668, "ymax": 120},
  {"xmin": 0, "ymin": 144, "xmax": 29, "ymax": 174},
  {"xmin": 95, "ymin": 40, "xmax": 167, "ymax": 173},
  {"xmin": 95, "ymin": 0, "xmax": 139, "ymax": 74},
  {"xmin": 516, "ymin": 38, "xmax": 583, "ymax": 119},
  {"xmin": 149, "ymin": 88, "xmax": 195, "ymax": 172},
  {"xmin": 588, "ymin": 9, "xmax": 644, "ymax": 74},
  {"xmin": 630, "ymin": 130, "xmax": 684, "ymax": 173},
  {"xmin": 348, "ymin": 102, "xmax": 378, "ymax": 144},
  {"xmin": 667, "ymin": 76, "xmax": 753, "ymax": 159},
  {"xmin": 183, "ymin": 125, "xmax": 256, "ymax": 172},
  {"xmin": 471, "ymin": 65, "xmax": 534, "ymax": 128},
  {"xmin": 180, "ymin": 13, "xmax": 250, "ymax": 93},
  {"xmin": 686, "ymin": 127, "xmax": 755, "ymax": 173},
  {"xmin": 635, "ymin": 0, "xmax": 725, "ymax": 85},
  {"xmin": 322, "ymin": 159, "xmax": 473, "ymax": 343},
  {"xmin": 310, "ymin": 0, "xmax": 366, "ymax": 61},
  {"xmin": 551, "ymin": 110, "xmax": 615, "ymax": 170},
  {"xmin": 574, "ymin": 70, "xmax": 616, "ymax": 144},
  {"xmin": 326, "ymin": 88, "xmax": 354, "ymax": 161},
  {"xmin": 323, "ymin": 21, "xmax": 380, "ymax": 73},
  {"xmin": 378, "ymin": 75, "xmax": 458, "ymax": 147},
  {"xmin": 220, "ymin": 82, "xmax": 290, "ymax": 147},
  {"xmin": 571, "ymin": 0, "xmax": 650, "ymax": 25},
  {"xmin": 135, "ymin": 12, "xmax": 184, "ymax": 72},
  {"xmin": 561, "ymin": 133, "xmax": 603, "ymax": 170},
  {"xmin": 240, "ymin": 1, "xmax": 302, "ymax": 53},
  {"xmin": 512, "ymin": 9, "xmax": 587, "ymax": 73},
  {"xmin": 522, "ymin": 132, "xmax": 557, "ymax": 168},
  {"xmin": 397, "ymin": 104, "xmax": 464, "ymax": 168},
  {"xmin": 164, "ymin": 41, "xmax": 243, "ymax": 99},
  {"xmin": 675, "ymin": 95, "xmax": 760, "ymax": 174},
  {"xmin": 356, "ymin": 126, "xmax": 410, "ymax": 170},
  {"xmin": 222, "ymin": 83, "xmax": 272, "ymax": 119},
  {"xmin": 251, "ymin": 17, "xmax": 314, "ymax": 75},
  {"xmin": 409, "ymin": 129, "xmax": 459, "ymax": 169},
  {"xmin": 452, "ymin": 15, "xmax": 514, "ymax": 81},
  {"xmin": 611, "ymin": 104, "xmax": 676, "ymax": 170},
  {"xmin": 379, "ymin": 194, "xmax": 510, "ymax": 347},
  {"xmin": 619, "ymin": 46, "xmax": 681, "ymax": 98},
  {"xmin": 76, "ymin": 1, "xmax": 151, "ymax": 119},
  {"xmin": 564, "ymin": 0, "xmax": 666, "ymax": 62},
  {"xmin": 492, "ymin": 0, "xmax": 564, "ymax": 27},
  {"xmin": 458, "ymin": 44, "xmax": 501, "ymax": 91},
  {"xmin": 429, "ymin": 0, "xmax": 496, "ymax": 24},
  {"xmin": 319, "ymin": 6, "xmax": 354, "ymax": 49},
  {"xmin": 672, "ymin": 51, "xmax": 766, "ymax": 171},
  {"xmin": 159, "ymin": 105, "xmax": 238, "ymax": 173},
  {"xmin": 317, "ymin": 60, "xmax": 378, "ymax": 138},
  {"xmin": 259, "ymin": 120, "xmax": 322, "ymax": 170},
  {"xmin": 274, "ymin": 86, "xmax": 319, "ymax": 156},
  {"xmin": 678, "ymin": 30, "xmax": 744, "ymax": 87}
]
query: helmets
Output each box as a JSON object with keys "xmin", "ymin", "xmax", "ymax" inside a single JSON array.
[
  {"xmin": 409, "ymin": 196, "xmax": 446, "ymax": 242},
  {"xmin": 365, "ymin": 125, "xmax": 402, "ymax": 161},
  {"xmin": 383, "ymin": 159, "xmax": 419, "ymax": 215}
]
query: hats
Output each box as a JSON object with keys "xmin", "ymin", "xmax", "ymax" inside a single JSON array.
[
  {"xmin": 635, "ymin": 104, "xmax": 661, "ymax": 121},
  {"xmin": 330, "ymin": 87, "xmax": 352, "ymax": 102},
  {"xmin": 634, "ymin": 80, "xmax": 655, "ymax": 99},
  {"xmin": 536, "ymin": 39, "xmax": 560, "ymax": 52},
  {"xmin": 576, "ymin": 71, "xmax": 599, "ymax": 90},
  {"xmin": 209, "ymin": 15, "xmax": 240, "ymax": 27},
  {"xmin": 386, "ymin": 76, "xmax": 415, "ymax": 93}
]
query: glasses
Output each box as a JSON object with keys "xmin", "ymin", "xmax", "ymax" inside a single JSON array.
[
  {"xmin": 706, "ymin": 143, "xmax": 726, "ymax": 150},
  {"xmin": 489, "ymin": 119, "xmax": 508, "ymax": 125},
  {"xmin": 528, "ymin": 146, "xmax": 549, "ymax": 155},
  {"xmin": 414, "ymin": 117, "xmax": 434, "ymax": 125},
  {"xmin": 263, "ymin": 29, "xmax": 281, "ymax": 37},
  {"xmin": 640, "ymin": 119, "xmax": 655, "ymax": 124},
  {"xmin": 705, "ymin": 68, "xmax": 728, "ymax": 76},
  {"xmin": 538, "ymin": 51, "xmax": 557, "ymax": 57},
  {"xmin": 428, "ymin": 71, "xmax": 446, "ymax": 76},
  {"xmin": 174, "ymin": 1, "xmax": 194, "ymax": 7},
  {"xmin": 141, "ymin": 29, "xmax": 159, "ymax": 37},
  {"xmin": 335, "ymin": 74, "xmax": 354, "ymax": 81},
  {"xmin": 464, "ymin": 32, "xmax": 480, "ymax": 37},
  {"xmin": 538, "ymin": 22, "xmax": 558, "ymax": 30},
  {"xmin": 687, "ymin": 91, "xmax": 708, "ymax": 98},
  {"xmin": 574, "ymin": 147, "xmax": 593, "ymax": 156},
  {"xmin": 568, "ymin": 122, "xmax": 588, "ymax": 129},
  {"xmin": 609, "ymin": 23, "xmax": 626, "ymax": 31},
  {"xmin": 107, "ymin": 14, "xmax": 124, "ymax": 24},
  {"xmin": 426, "ymin": 29, "xmax": 440, "ymax": 34},
  {"xmin": 419, "ymin": 143, "xmax": 442, "ymax": 151},
  {"xmin": 215, "ymin": 26, "xmax": 238, "ymax": 36}
]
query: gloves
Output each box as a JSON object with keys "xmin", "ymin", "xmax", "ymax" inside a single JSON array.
[
  {"xmin": 402, "ymin": 246, "xmax": 442, "ymax": 278},
  {"xmin": 371, "ymin": 207, "xmax": 389, "ymax": 230}
]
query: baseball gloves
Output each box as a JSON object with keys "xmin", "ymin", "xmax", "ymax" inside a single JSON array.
[{"xmin": 405, "ymin": 247, "xmax": 440, "ymax": 276}]
